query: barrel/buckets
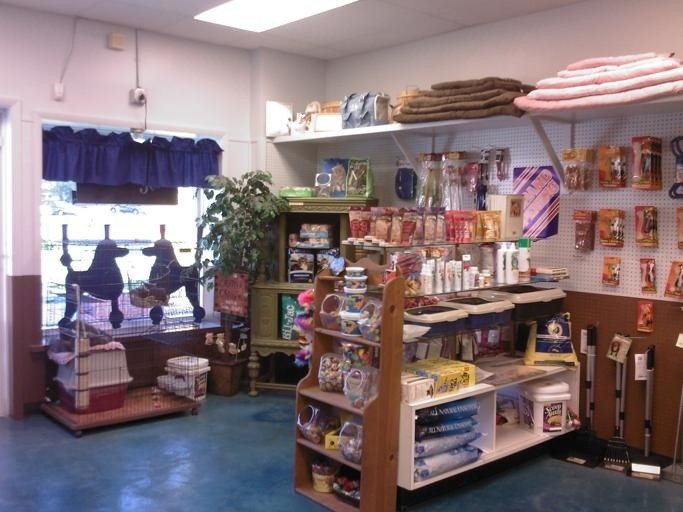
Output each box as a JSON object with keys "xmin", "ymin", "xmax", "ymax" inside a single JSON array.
[
  {"xmin": 518, "ymin": 379, "xmax": 572, "ymax": 433},
  {"xmin": 165, "ymin": 356, "xmax": 211, "ymax": 404}
]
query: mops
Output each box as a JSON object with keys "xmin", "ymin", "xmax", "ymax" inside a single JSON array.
[{"xmin": 630, "ymin": 344, "xmax": 662, "ymax": 481}]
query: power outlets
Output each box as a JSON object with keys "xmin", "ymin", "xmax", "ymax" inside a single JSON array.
[{"xmin": 128, "ymin": 88, "xmax": 146, "ymax": 106}]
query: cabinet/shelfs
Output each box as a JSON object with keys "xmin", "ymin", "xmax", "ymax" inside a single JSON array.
[
  {"xmin": 247, "ymin": 195, "xmax": 378, "ymax": 397},
  {"xmin": 292, "ymin": 238, "xmax": 581, "ymax": 512}
]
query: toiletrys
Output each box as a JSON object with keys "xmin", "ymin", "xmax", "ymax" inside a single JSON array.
[
  {"xmin": 419, "ymin": 254, "xmax": 477, "ymax": 298},
  {"xmin": 495, "ymin": 237, "xmax": 531, "ymax": 288}
]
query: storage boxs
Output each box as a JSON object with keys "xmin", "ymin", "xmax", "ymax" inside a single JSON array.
[
  {"xmin": 485, "ymin": 192, "xmax": 525, "ymax": 237},
  {"xmin": 402, "ymin": 305, "xmax": 468, "ymax": 337},
  {"xmin": 310, "ymin": 113, "xmax": 343, "ymax": 134},
  {"xmin": 52, "ymin": 342, "xmax": 133, "ymax": 413},
  {"xmin": 439, "ymin": 297, "xmax": 516, "ymax": 327},
  {"xmin": 481, "ymin": 284, "xmax": 567, "ymax": 321}
]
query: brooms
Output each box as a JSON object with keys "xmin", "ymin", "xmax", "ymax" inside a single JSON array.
[
  {"xmin": 662, "ymin": 380, "xmax": 683, "ymax": 483},
  {"xmin": 602, "ymin": 354, "xmax": 630, "ymax": 464}
]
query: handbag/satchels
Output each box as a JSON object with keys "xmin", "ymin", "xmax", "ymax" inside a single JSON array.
[{"xmin": 341, "ymin": 92, "xmax": 390, "ymax": 129}]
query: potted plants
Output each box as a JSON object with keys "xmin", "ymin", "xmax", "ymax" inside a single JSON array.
[{"xmin": 193, "ymin": 168, "xmax": 291, "ymax": 397}]
